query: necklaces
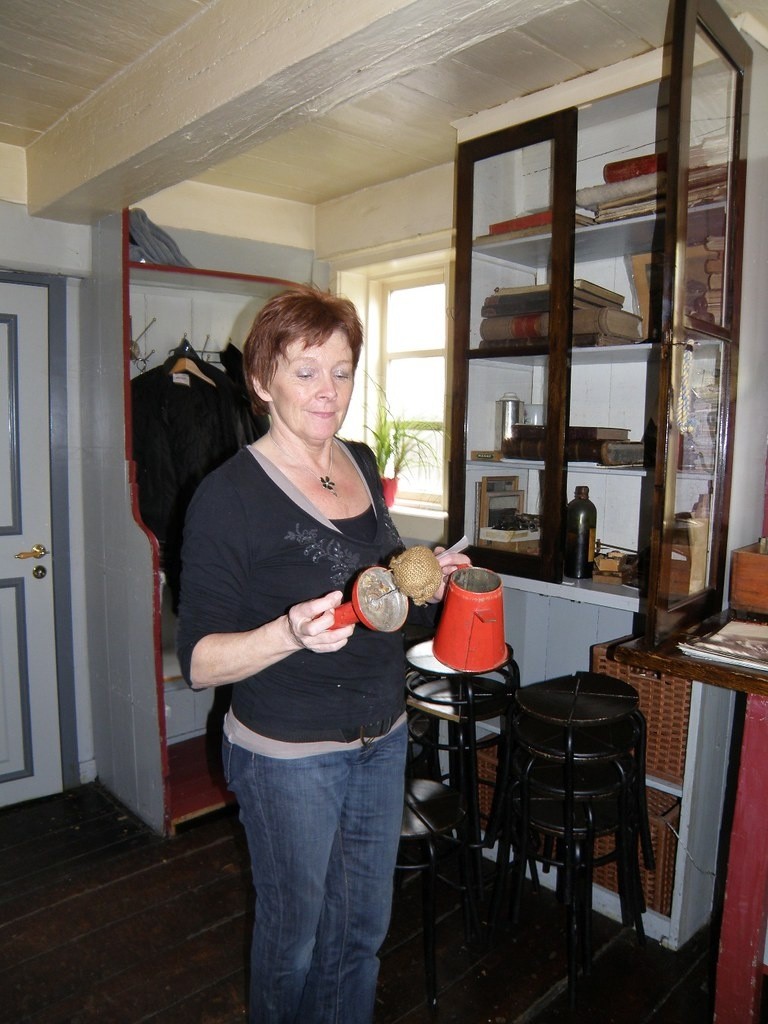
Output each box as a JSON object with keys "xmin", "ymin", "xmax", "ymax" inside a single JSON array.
[{"xmin": 268, "ymin": 430, "xmax": 339, "ymax": 496}]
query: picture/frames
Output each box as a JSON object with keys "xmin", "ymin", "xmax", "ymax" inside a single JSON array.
[{"xmin": 479, "ymin": 474, "xmax": 524, "ymax": 528}]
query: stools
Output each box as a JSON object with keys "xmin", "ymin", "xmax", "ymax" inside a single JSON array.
[{"xmin": 392, "ymin": 640, "xmax": 656, "ymax": 1023}]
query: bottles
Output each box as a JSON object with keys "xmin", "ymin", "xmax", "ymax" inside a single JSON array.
[
  {"xmin": 495, "ymin": 391, "xmax": 524, "ymax": 452},
  {"xmin": 564, "ymin": 486, "xmax": 597, "ymax": 579}
]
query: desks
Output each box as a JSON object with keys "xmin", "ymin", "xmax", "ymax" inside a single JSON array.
[{"xmin": 614, "ymin": 609, "xmax": 768, "ymax": 1024}]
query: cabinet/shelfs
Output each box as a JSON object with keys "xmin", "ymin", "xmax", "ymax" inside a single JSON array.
[
  {"xmin": 89, "ymin": 205, "xmax": 302, "ymax": 838},
  {"xmin": 437, "ymin": 0, "xmax": 768, "ymax": 952}
]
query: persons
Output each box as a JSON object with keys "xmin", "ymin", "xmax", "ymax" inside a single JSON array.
[{"xmin": 177, "ymin": 282, "xmax": 470, "ymax": 1024}]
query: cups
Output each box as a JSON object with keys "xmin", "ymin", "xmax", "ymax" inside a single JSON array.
[{"xmin": 523, "ymin": 404, "xmax": 545, "ymax": 426}]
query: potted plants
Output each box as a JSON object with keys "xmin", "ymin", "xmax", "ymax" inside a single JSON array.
[{"xmin": 358, "ymin": 370, "xmax": 451, "ymax": 508}]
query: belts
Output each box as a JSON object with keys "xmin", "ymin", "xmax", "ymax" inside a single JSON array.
[{"xmin": 338, "ymin": 700, "xmax": 407, "ymax": 747}]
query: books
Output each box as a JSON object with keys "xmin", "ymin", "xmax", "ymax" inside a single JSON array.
[
  {"xmin": 479, "ymin": 235, "xmax": 725, "ymax": 350},
  {"xmin": 675, "ymin": 617, "xmax": 768, "ymax": 671},
  {"xmin": 503, "ymin": 424, "xmax": 644, "ymax": 466},
  {"xmin": 683, "ymin": 384, "xmax": 718, "ymax": 472},
  {"xmin": 472, "ymin": 143, "xmax": 727, "ymax": 247}
]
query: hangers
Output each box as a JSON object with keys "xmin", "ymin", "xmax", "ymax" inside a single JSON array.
[{"xmin": 169, "ymin": 338, "xmax": 217, "ymax": 387}]
